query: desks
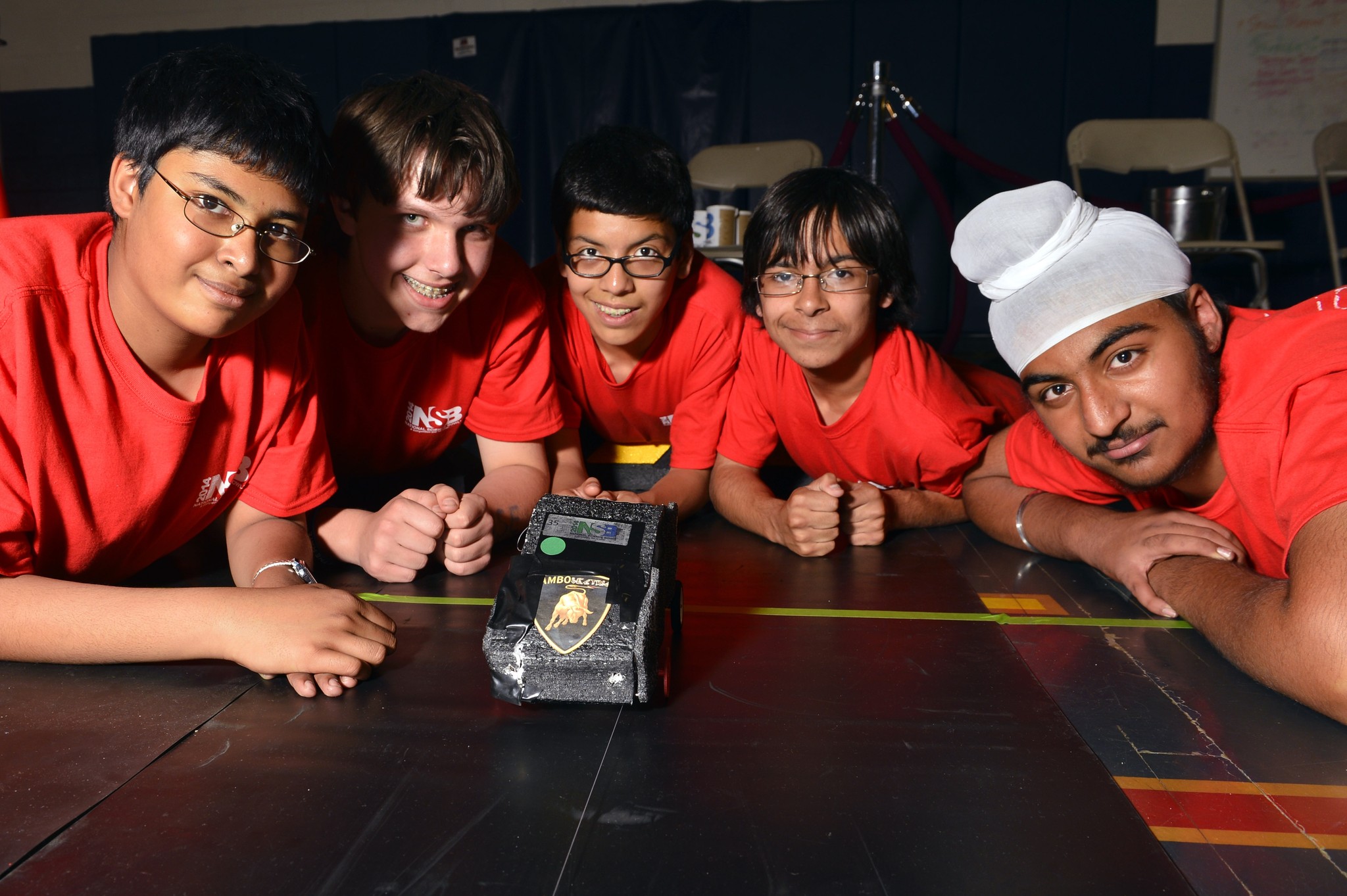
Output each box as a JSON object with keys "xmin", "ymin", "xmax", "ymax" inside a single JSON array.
[{"xmin": 0, "ymin": 509, "xmax": 1346, "ymax": 896}]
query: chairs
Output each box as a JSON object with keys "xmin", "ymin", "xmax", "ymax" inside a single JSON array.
[
  {"xmin": 1067, "ymin": 118, "xmax": 1283, "ymax": 310},
  {"xmin": 1313, "ymin": 121, "xmax": 1347, "ymax": 289},
  {"xmin": 686, "ymin": 141, "xmax": 823, "ymax": 264}
]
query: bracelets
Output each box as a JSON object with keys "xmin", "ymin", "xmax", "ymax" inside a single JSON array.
[{"xmin": 1015, "ymin": 489, "xmax": 1049, "ymax": 554}]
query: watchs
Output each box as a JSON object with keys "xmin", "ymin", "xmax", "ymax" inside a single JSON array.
[{"xmin": 251, "ymin": 556, "xmax": 318, "ymax": 589}]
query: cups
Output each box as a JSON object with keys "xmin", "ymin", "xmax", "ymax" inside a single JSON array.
[{"xmin": 691, "ymin": 203, "xmax": 754, "ymax": 247}]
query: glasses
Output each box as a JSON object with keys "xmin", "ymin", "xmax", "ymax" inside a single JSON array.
[
  {"xmin": 561, "ymin": 242, "xmax": 680, "ymax": 279},
  {"xmin": 144, "ymin": 160, "xmax": 316, "ymax": 264},
  {"xmin": 751, "ymin": 267, "xmax": 877, "ymax": 296}
]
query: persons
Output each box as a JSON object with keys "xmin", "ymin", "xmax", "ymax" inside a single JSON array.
[
  {"xmin": 962, "ymin": 206, "xmax": 1346, "ymax": 726},
  {"xmin": 291, "ymin": 73, "xmax": 570, "ymax": 585},
  {"xmin": 0, "ymin": 68, "xmax": 396, "ymax": 703},
  {"xmin": 707, "ymin": 165, "xmax": 994, "ymax": 559},
  {"xmin": 531, "ymin": 133, "xmax": 748, "ymax": 515}
]
